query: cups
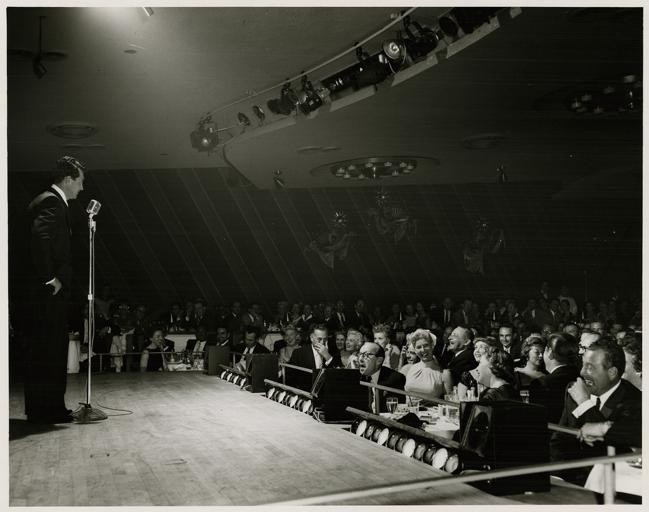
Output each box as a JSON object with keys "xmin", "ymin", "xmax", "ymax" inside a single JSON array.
[
  {"xmin": 438, "ymin": 395, "xmax": 459, "ymax": 421},
  {"xmin": 407, "ymin": 400, "xmax": 419, "ymax": 415}
]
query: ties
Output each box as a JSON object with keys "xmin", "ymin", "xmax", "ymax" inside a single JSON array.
[
  {"xmin": 340, "ymin": 312, "xmax": 345, "ymax": 326},
  {"xmin": 446, "ymin": 309, "xmax": 449, "ymax": 323},
  {"xmin": 595, "ymin": 397, "xmax": 600, "ymax": 411},
  {"xmin": 244, "ymin": 347, "xmax": 250, "ymax": 360}
]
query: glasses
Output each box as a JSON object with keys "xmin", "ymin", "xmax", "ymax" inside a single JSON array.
[
  {"xmin": 357, "ymin": 351, "xmax": 376, "ymax": 359},
  {"xmin": 578, "ymin": 343, "xmax": 586, "ymax": 349}
]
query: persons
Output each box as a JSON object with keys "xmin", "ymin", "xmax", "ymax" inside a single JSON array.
[{"xmin": 23, "ymin": 154, "xmax": 85, "ymax": 425}]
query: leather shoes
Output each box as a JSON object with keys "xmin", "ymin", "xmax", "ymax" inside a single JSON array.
[
  {"xmin": 64, "ymin": 409, "xmax": 72, "ymax": 414},
  {"xmin": 29, "ymin": 415, "xmax": 73, "ymax": 423}
]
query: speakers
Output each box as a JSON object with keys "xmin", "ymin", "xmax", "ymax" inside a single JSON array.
[
  {"xmin": 205, "ymin": 346, "xmax": 230, "ymax": 375},
  {"xmin": 311, "ymin": 369, "xmax": 360, "ymax": 424},
  {"xmin": 459, "ymin": 400, "xmax": 549, "ymax": 496},
  {"xmin": 246, "ymin": 354, "xmax": 278, "ymax": 392}
]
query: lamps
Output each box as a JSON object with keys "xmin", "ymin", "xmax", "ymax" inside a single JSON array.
[
  {"xmin": 495, "ymin": 164, "xmax": 509, "ymax": 185},
  {"xmin": 273, "ymin": 170, "xmax": 289, "ymax": 192},
  {"xmin": 186, "ymin": 6, "xmax": 529, "ymax": 155}
]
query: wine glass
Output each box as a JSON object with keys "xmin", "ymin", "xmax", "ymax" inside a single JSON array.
[{"xmin": 386, "ymin": 397, "xmax": 398, "ymax": 421}]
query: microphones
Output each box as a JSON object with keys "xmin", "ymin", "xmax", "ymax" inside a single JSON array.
[{"xmin": 86, "ymin": 199, "xmax": 102, "ymax": 219}]
